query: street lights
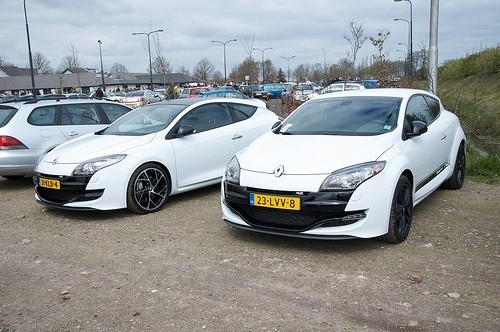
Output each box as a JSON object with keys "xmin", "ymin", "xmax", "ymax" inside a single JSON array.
[
  {"xmin": 392, "ymin": 0, "xmax": 415, "ymax": 79},
  {"xmin": 280, "ymin": 55, "xmax": 296, "ymax": 82},
  {"xmin": 253, "ymin": 47, "xmax": 274, "ymax": 85},
  {"xmin": 132, "ymin": 29, "xmax": 164, "ymax": 91},
  {"xmin": 211, "ymin": 39, "xmax": 237, "ymax": 81}
]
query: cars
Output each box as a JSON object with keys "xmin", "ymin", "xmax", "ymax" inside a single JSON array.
[
  {"xmin": 0, "ymin": 97, "xmax": 134, "ymax": 180},
  {"xmin": 47, "ymin": 76, "xmax": 400, "ymax": 109},
  {"xmin": 221, "ymin": 88, "xmax": 466, "ymax": 244},
  {"xmin": 33, "ymin": 98, "xmax": 284, "ymax": 215}
]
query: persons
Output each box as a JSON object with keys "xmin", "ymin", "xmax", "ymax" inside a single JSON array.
[
  {"xmin": 281, "ymin": 89, "xmax": 288, "ymax": 106},
  {"xmin": 308, "ymin": 88, "xmax": 320, "ymax": 99}
]
